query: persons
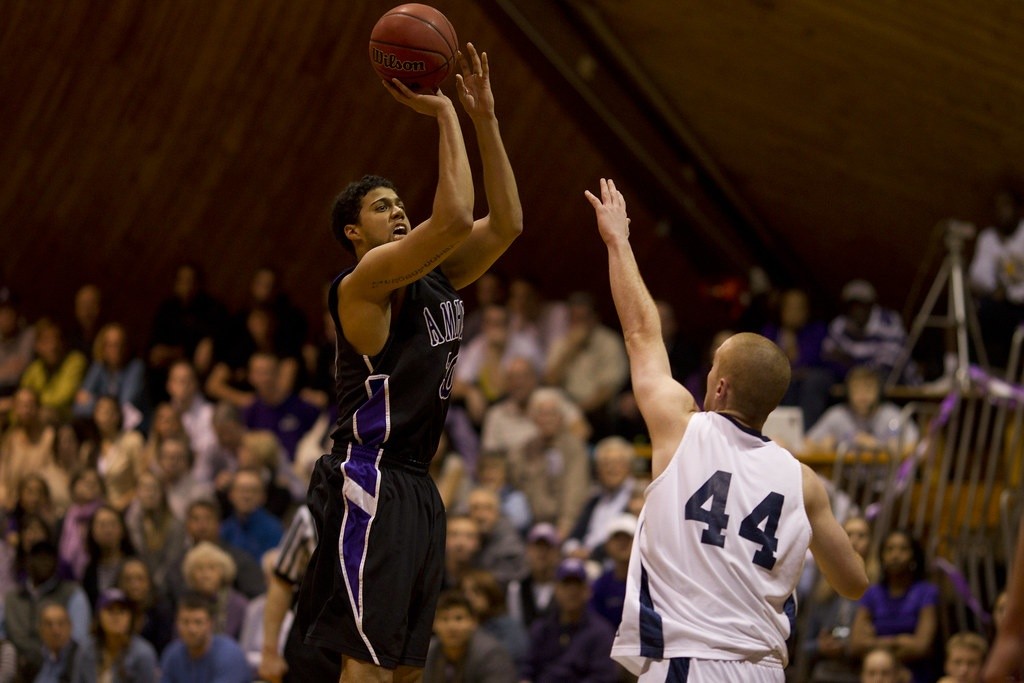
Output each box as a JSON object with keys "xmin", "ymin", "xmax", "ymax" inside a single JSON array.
[
  {"xmin": 0, "ymin": 260, "xmax": 1024, "ymax": 683},
  {"xmin": 306, "ymin": 42, "xmax": 523, "ymax": 683},
  {"xmin": 584, "ymin": 178, "xmax": 870, "ymax": 683}
]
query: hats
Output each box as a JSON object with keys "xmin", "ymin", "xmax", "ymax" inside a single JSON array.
[{"xmin": 183, "ymin": 541, "xmax": 236, "ymax": 589}]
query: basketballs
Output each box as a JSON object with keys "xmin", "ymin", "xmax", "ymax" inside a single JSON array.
[{"xmin": 371, "ymin": 3, "xmax": 459, "ymax": 95}]
